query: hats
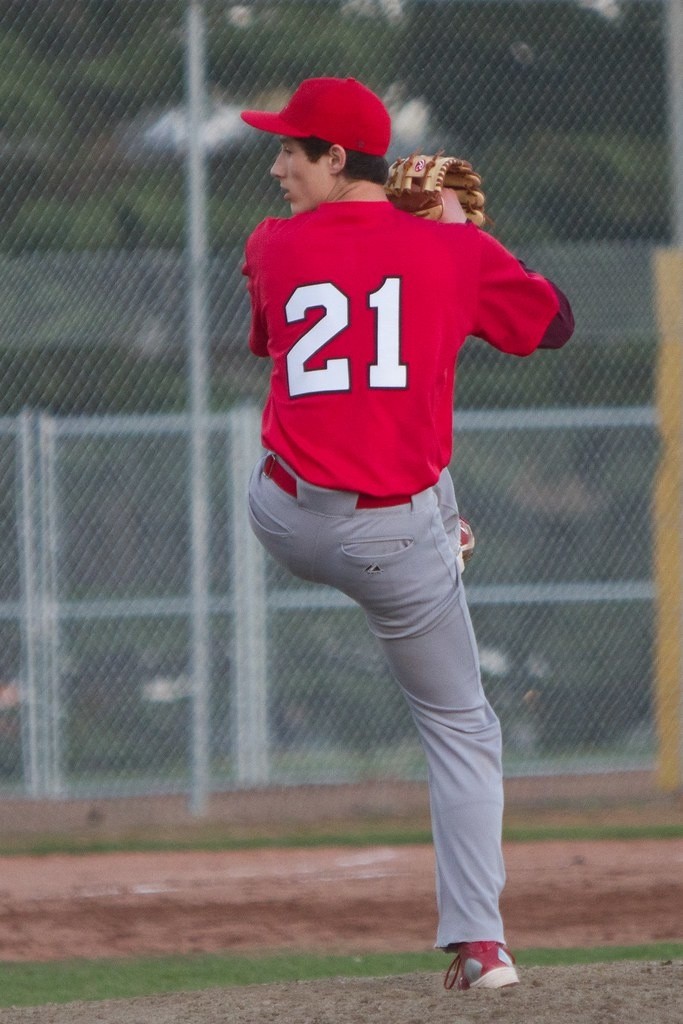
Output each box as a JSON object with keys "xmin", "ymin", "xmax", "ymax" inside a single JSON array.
[{"xmin": 240, "ymin": 76, "xmax": 391, "ymax": 157}]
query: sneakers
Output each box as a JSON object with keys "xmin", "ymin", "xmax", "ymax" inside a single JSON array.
[
  {"xmin": 457, "ymin": 516, "xmax": 475, "ymax": 559},
  {"xmin": 443, "ymin": 941, "xmax": 520, "ymax": 991}
]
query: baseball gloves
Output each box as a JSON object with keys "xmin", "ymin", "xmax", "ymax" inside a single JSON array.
[{"xmin": 382, "ymin": 144, "xmax": 497, "ymax": 231}]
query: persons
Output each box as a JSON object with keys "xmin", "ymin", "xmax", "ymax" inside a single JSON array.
[
  {"xmin": 382, "ymin": 78, "xmax": 430, "ymax": 172},
  {"xmin": 240, "ymin": 78, "xmax": 575, "ymax": 988},
  {"xmin": 150, "ymin": 73, "xmax": 289, "ymax": 148}
]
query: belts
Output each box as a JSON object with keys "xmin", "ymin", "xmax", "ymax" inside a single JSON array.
[{"xmin": 260, "ymin": 454, "xmax": 411, "ymax": 508}]
